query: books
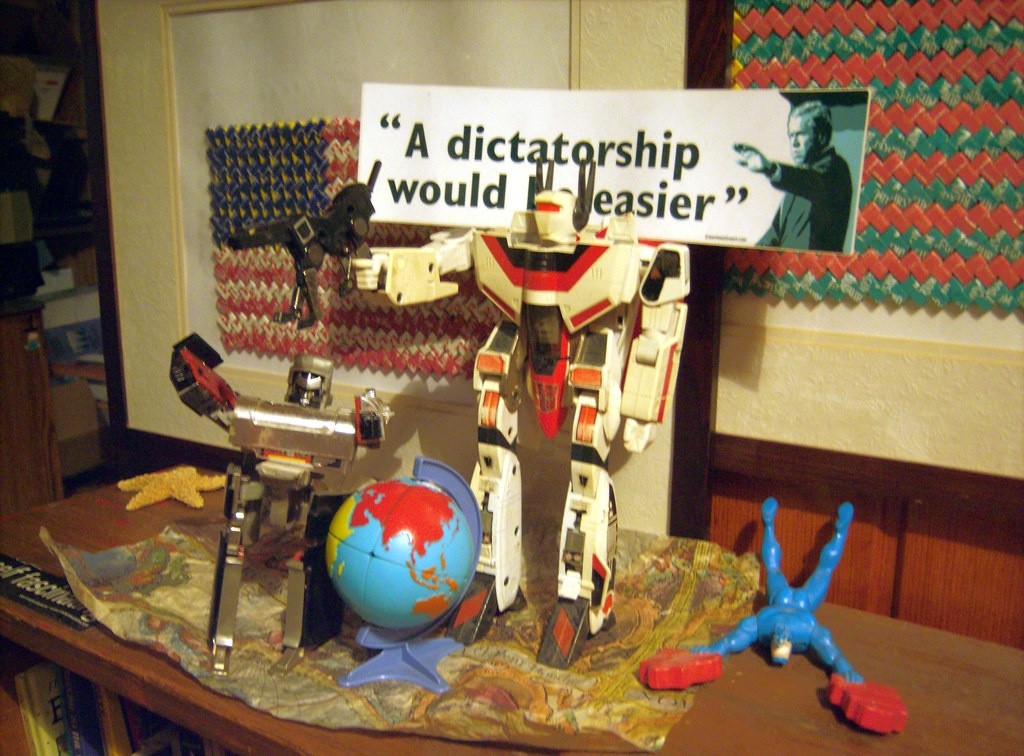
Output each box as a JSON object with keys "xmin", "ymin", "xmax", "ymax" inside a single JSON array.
[{"xmin": 13, "ymin": 656, "xmax": 237, "ymax": 756}]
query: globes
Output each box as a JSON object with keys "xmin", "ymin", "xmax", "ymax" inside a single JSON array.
[{"xmin": 323, "ymin": 455, "xmax": 482, "ymax": 694}]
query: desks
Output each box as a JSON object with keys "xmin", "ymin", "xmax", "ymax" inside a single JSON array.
[{"xmin": 0, "ymin": 465, "xmax": 1024, "ymax": 756}]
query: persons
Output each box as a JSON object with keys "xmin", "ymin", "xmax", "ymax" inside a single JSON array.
[{"xmin": 731, "ymin": 100, "xmax": 852, "ymax": 252}]
